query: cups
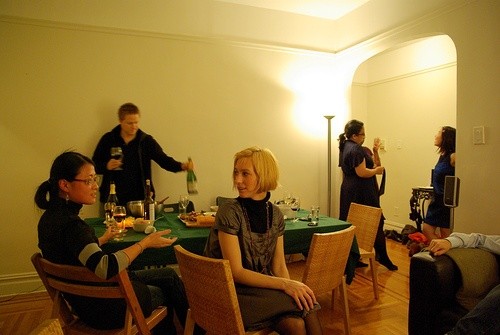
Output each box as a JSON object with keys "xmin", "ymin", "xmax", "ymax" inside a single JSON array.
[{"xmin": 311, "ymin": 206, "xmax": 320, "ymax": 225}]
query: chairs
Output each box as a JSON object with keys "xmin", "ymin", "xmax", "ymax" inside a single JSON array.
[
  {"xmin": 287, "ymin": 226, "xmax": 356, "ymax": 335},
  {"xmin": 31, "ymin": 252, "xmax": 167, "ymax": 335},
  {"xmin": 348, "ymin": 203, "xmax": 382, "ymax": 299},
  {"xmin": 174, "ymin": 245, "xmax": 274, "ymax": 335}
]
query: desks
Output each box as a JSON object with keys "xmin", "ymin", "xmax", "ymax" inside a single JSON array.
[
  {"xmin": 85, "ymin": 208, "xmax": 368, "ymax": 285},
  {"xmin": 409, "ymin": 186, "xmax": 433, "ymax": 232}
]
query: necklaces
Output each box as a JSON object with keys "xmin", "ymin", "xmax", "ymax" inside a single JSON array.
[{"xmin": 240, "ymin": 198, "xmax": 269, "ymax": 274}]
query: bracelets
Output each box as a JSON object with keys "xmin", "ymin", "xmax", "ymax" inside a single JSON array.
[{"xmin": 137, "ymin": 242, "xmax": 144, "ymax": 255}]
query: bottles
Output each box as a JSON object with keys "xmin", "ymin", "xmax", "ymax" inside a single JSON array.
[
  {"xmin": 144, "ymin": 179, "xmax": 155, "ymax": 226},
  {"xmin": 186, "ymin": 158, "xmax": 198, "ymax": 194},
  {"xmin": 106, "ymin": 183, "xmax": 118, "ymax": 218}
]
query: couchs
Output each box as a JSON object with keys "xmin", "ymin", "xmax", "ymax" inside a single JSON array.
[{"xmin": 408, "ymin": 248, "xmax": 500, "ymax": 335}]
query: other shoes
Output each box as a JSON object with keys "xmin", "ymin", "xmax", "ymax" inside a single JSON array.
[
  {"xmin": 375, "ymin": 254, "xmax": 398, "ymax": 271},
  {"xmin": 355, "ymin": 261, "xmax": 368, "ymax": 268}
]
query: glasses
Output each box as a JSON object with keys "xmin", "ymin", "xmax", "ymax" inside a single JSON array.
[
  {"xmin": 357, "ymin": 132, "xmax": 365, "ymax": 136},
  {"xmin": 69, "ymin": 174, "xmax": 101, "ymax": 186}
]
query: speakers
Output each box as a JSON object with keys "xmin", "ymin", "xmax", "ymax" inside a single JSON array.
[{"xmin": 443, "ymin": 176, "xmax": 458, "ymax": 208}]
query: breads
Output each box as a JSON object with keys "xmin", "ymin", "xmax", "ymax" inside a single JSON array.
[{"xmin": 196, "ymin": 214, "xmax": 215, "ymax": 224}]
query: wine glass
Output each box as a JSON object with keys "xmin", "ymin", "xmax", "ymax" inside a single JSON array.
[
  {"xmin": 179, "ymin": 194, "xmax": 190, "ymax": 213},
  {"xmin": 283, "ymin": 192, "xmax": 299, "ymax": 223},
  {"xmin": 113, "ymin": 206, "xmax": 126, "ymax": 240},
  {"xmin": 111, "ymin": 146, "xmax": 123, "ymax": 170}
]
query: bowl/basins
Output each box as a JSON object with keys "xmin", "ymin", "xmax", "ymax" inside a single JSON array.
[
  {"xmin": 132, "ymin": 221, "xmax": 154, "ymax": 232},
  {"xmin": 127, "ymin": 200, "xmax": 144, "ymax": 217},
  {"xmin": 128, "ymin": 200, "xmax": 163, "ymax": 218}
]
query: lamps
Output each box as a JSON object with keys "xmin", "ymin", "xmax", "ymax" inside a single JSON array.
[{"xmin": 324, "ymin": 114, "xmax": 335, "ymax": 218}]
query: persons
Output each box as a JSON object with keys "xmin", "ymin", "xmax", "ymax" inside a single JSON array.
[
  {"xmin": 35, "ymin": 152, "xmax": 203, "ymax": 335},
  {"xmin": 203, "ymin": 145, "xmax": 324, "ymax": 335},
  {"xmin": 428, "ymin": 232, "xmax": 500, "ymax": 335},
  {"xmin": 92, "ymin": 102, "xmax": 194, "ymax": 218},
  {"xmin": 423, "ymin": 126, "xmax": 457, "ymax": 240},
  {"xmin": 338, "ymin": 120, "xmax": 399, "ymax": 271}
]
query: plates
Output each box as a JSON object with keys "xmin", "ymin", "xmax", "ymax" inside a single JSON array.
[{"xmin": 176, "ymin": 212, "xmax": 217, "ymax": 227}]
